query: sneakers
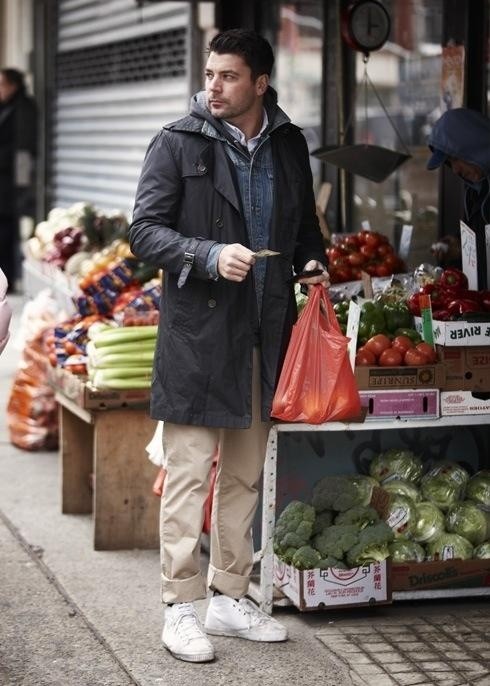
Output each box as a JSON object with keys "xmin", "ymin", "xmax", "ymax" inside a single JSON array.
[
  {"xmin": 205, "ymin": 594, "xmax": 290, "ymax": 644},
  {"xmin": 160, "ymin": 600, "xmax": 217, "ymax": 664}
]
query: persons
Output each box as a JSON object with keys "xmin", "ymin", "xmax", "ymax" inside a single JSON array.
[
  {"xmin": 425, "ymin": 105, "xmax": 490, "ymax": 293},
  {"xmin": 127, "ymin": 19, "xmax": 334, "ymax": 665},
  {"xmin": 0, "ymin": 65, "xmax": 41, "ymax": 295}
]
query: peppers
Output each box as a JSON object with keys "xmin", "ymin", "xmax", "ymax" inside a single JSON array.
[{"xmin": 332, "ymin": 268, "xmax": 490, "ymax": 340}]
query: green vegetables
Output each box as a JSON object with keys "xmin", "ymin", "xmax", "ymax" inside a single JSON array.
[{"xmin": 84, "ymin": 320, "xmax": 158, "ymax": 392}]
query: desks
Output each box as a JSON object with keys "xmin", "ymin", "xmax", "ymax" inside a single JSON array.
[
  {"xmin": 54, "ymin": 392, "xmax": 174, "ymax": 550},
  {"xmin": 259, "ymin": 411, "xmax": 490, "ymax": 610}
]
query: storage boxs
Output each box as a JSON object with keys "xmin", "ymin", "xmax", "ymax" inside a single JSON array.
[
  {"xmin": 390, "ymin": 559, "xmax": 490, "ymax": 590},
  {"xmin": 353, "ymin": 346, "xmax": 490, "ymax": 415},
  {"xmin": 274, "ymin": 554, "xmax": 392, "ymax": 614},
  {"xmin": 47, "ymin": 368, "xmax": 153, "ymax": 410}
]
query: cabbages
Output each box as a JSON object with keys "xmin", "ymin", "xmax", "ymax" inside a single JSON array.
[{"xmin": 351, "ymin": 449, "xmax": 490, "ymax": 563}]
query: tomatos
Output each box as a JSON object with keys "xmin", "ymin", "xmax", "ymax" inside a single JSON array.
[
  {"xmin": 326, "ymin": 229, "xmax": 404, "ymax": 283},
  {"xmin": 355, "ymin": 334, "xmax": 437, "ymax": 366}
]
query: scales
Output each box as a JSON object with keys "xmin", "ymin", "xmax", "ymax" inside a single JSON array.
[{"xmin": 310, "ymin": 0, "xmax": 413, "ymax": 183}]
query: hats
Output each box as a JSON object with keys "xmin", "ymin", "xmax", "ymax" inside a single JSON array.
[{"xmin": 425, "ymin": 149, "xmax": 447, "ymax": 170}]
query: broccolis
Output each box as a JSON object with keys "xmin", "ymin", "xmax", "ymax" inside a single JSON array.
[{"xmin": 273, "ymin": 472, "xmax": 394, "ymax": 569}]
